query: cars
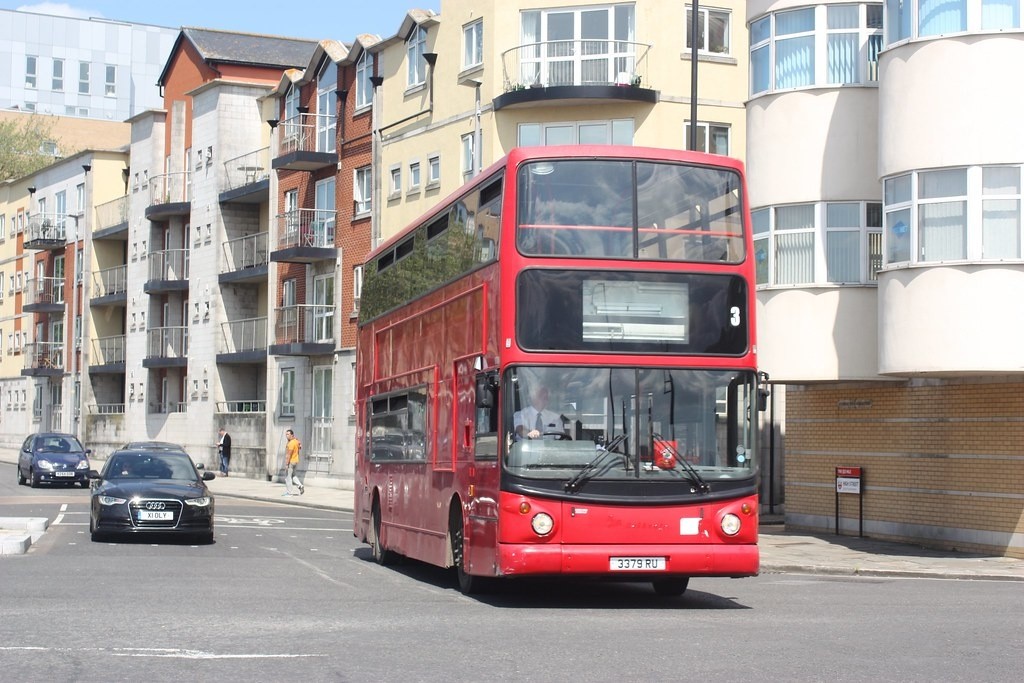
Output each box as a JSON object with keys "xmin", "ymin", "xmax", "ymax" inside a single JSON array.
[
  {"xmin": 122, "ymin": 440, "xmax": 205, "ymax": 470},
  {"xmin": 86, "ymin": 448, "xmax": 216, "ymax": 543},
  {"xmin": 17, "ymin": 432, "xmax": 93, "ymax": 488}
]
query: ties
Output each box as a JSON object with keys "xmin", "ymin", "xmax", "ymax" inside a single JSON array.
[{"xmin": 535, "ymin": 412, "xmax": 545, "ymax": 438}]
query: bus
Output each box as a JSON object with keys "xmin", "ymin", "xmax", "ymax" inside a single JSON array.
[{"xmin": 350, "ymin": 143, "xmax": 762, "ymax": 596}]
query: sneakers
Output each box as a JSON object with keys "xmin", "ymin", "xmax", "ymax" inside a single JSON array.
[
  {"xmin": 281, "ymin": 491, "xmax": 294, "ymax": 497},
  {"xmin": 299, "ymin": 485, "xmax": 304, "ymax": 495}
]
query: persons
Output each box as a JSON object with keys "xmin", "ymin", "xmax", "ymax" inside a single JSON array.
[
  {"xmin": 216, "ymin": 428, "xmax": 231, "ymax": 477},
  {"xmin": 513, "ymin": 388, "xmax": 565, "ymax": 442},
  {"xmin": 281, "ymin": 430, "xmax": 304, "ymax": 497},
  {"xmin": 117, "ymin": 460, "xmax": 138, "ymax": 477},
  {"xmin": 36, "ymin": 438, "xmax": 49, "ymax": 450}
]
